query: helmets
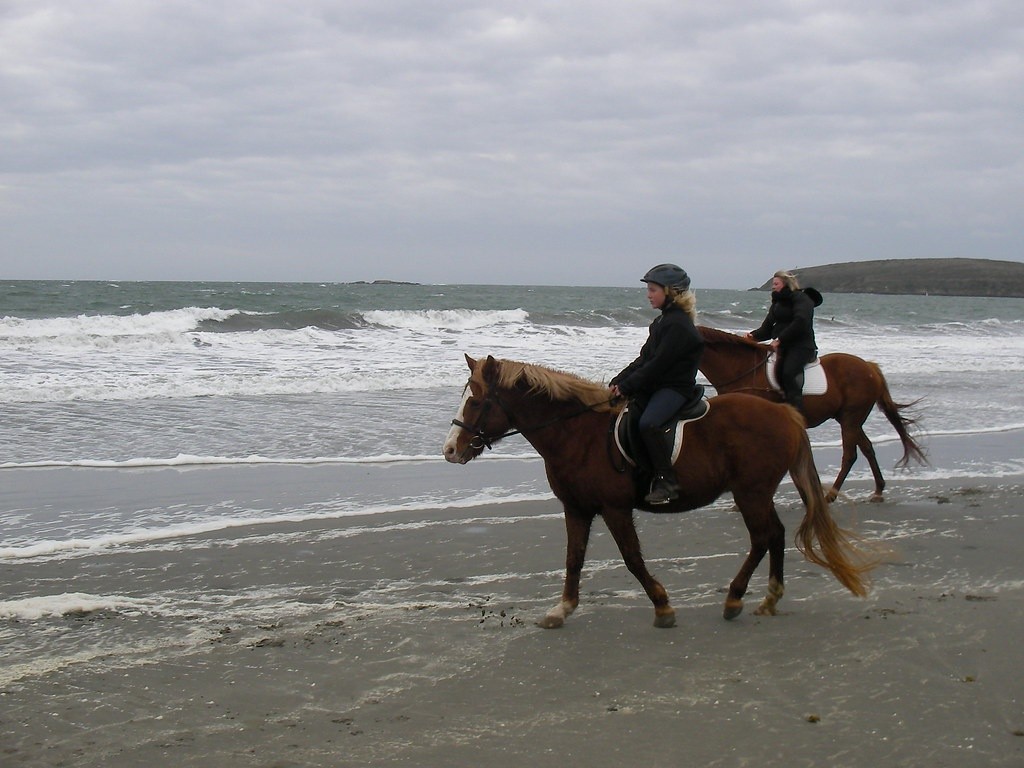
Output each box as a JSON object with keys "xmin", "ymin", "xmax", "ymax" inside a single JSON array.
[{"xmin": 639, "ymin": 263, "xmax": 692, "ymax": 302}]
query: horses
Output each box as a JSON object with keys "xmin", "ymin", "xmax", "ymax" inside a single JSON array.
[
  {"xmin": 693, "ymin": 326, "xmax": 931, "ymax": 503},
  {"xmin": 441, "ymin": 353, "xmax": 898, "ymax": 629}
]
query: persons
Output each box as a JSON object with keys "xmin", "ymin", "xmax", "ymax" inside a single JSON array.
[
  {"xmin": 742, "ymin": 269, "xmax": 817, "ymax": 410},
  {"xmin": 607, "ymin": 263, "xmax": 703, "ymax": 504}
]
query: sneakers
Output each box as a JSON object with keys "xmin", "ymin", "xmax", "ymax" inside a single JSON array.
[{"xmin": 644, "ymin": 480, "xmax": 685, "ymax": 502}]
